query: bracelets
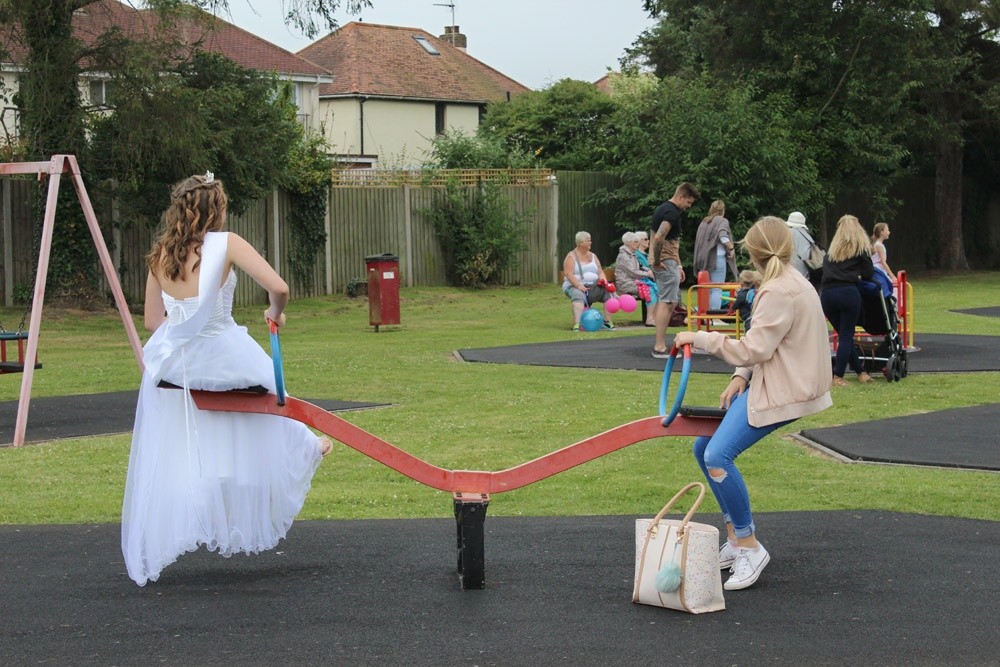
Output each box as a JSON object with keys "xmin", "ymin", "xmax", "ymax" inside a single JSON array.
[
  {"xmin": 728, "ymin": 247, "xmax": 734, "ymax": 251},
  {"xmin": 678, "ymin": 265, "xmax": 683, "ymax": 269}
]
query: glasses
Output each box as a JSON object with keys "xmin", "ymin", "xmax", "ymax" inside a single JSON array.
[{"xmin": 641, "ymin": 238, "xmax": 648, "ymax": 241}]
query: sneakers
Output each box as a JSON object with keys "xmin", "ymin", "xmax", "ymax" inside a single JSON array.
[
  {"xmin": 719, "ymin": 541, "xmax": 740, "ymax": 570},
  {"xmin": 723, "ymin": 541, "xmax": 771, "ymax": 591},
  {"xmin": 601, "ymin": 320, "xmax": 616, "ymax": 330},
  {"xmin": 571, "ymin": 323, "xmax": 579, "ymax": 331},
  {"xmin": 652, "ymin": 347, "xmax": 683, "ymax": 359}
]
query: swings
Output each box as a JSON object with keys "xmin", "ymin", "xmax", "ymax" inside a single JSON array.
[{"xmin": 0, "ymin": 181, "xmax": 43, "ymax": 374}]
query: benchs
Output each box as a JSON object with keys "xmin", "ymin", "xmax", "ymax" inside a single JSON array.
[{"xmin": 560, "ymin": 268, "xmax": 648, "ymax": 325}]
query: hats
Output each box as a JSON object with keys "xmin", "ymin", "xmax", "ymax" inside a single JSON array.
[{"xmin": 787, "ymin": 212, "xmax": 809, "ymax": 230}]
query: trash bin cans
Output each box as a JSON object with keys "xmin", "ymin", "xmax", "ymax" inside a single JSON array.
[{"xmin": 365, "ymin": 252, "xmax": 401, "ymax": 325}]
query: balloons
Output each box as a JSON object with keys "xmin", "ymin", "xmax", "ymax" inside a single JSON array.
[
  {"xmin": 620, "ymin": 294, "xmax": 637, "ymax": 312},
  {"xmin": 578, "ymin": 307, "xmax": 604, "ymax": 332},
  {"xmin": 605, "ymin": 298, "xmax": 620, "ymax": 313}
]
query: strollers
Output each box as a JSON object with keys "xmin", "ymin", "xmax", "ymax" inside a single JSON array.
[{"xmin": 852, "ymin": 266, "xmax": 908, "ymax": 384}]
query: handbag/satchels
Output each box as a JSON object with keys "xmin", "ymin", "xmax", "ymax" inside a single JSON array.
[
  {"xmin": 632, "ymin": 482, "xmax": 726, "ymax": 614},
  {"xmin": 808, "ymin": 267, "xmax": 824, "ymax": 291},
  {"xmin": 668, "ymin": 307, "xmax": 687, "ymax": 326}
]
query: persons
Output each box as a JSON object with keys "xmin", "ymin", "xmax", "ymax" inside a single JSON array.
[
  {"xmin": 649, "ymin": 182, "xmax": 700, "ymax": 358},
  {"xmin": 562, "ymin": 231, "xmax": 617, "ymax": 332},
  {"xmin": 615, "ymin": 230, "xmax": 658, "ymax": 327},
  {"xmin": 693, "ymin": 200, "xmax": 738, "ymax": 325},
  {"xmin": 120, "ymin": 170, "xmax": 333, "ymax": 587},
  {"xmin": 785, "ymin": 212, "xmax": 898, "ymax": 386},
  {"xmin": 673, "ymin": 216, "xmax": 833, "ymax": 590},
  {"xmin": 732, "ymin": 270, "xmax": 761, "ymax": 335}
]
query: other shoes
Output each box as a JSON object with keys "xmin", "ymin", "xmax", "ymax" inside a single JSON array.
[
  {"xmin": 907, "ymin": 346, "xmax": 921, "ymax": 353},
  {"xmin": 320, "ymin": 438, "xmax": 333, "ymax": 457},
  {"xmin": 833, "ymin": 376, "xmax": 849, "ymax": 386},
  {"xmin": 858, "ymin": 373, "xmax": 873, "ymax": 382}
]
